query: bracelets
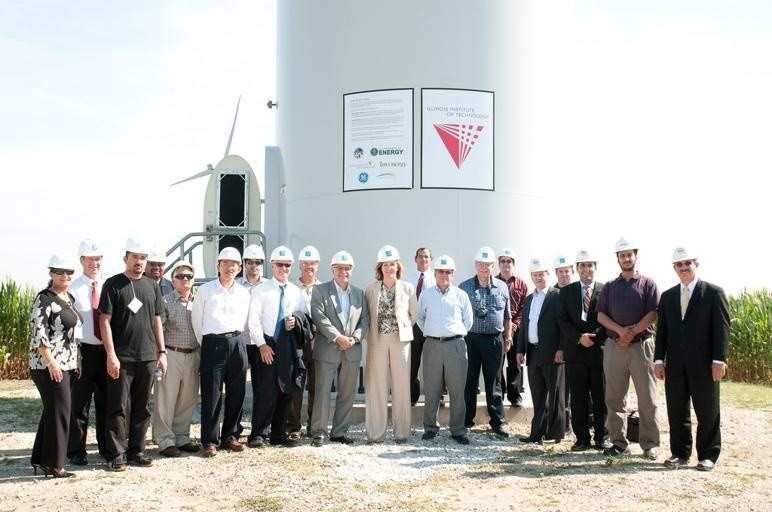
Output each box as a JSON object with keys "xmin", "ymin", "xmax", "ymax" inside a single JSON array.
[
  {"xmin": 505, "ymin": 336, "xmax": 513, "ymax": 341},
  {"xmin": 77, "ymin": 355, "xmax": 82, "ymax": 359},
  {"xmin": 46, "ymin": 358, "xmax": 55, "ymax": 366}
]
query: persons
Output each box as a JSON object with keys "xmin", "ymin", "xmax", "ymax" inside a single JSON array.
[
  {"xmin": 97, "ymin": 237, "xmax": 168, "ymax": 470},
  {"xmin": 30, "ymin": 254, "xmax": 84, "ymax": 476},
  {"xmin": 236, "ymin": 244, "xmax": 269, "ymax": 440},
  {"xmin": 497, "ymin": 249, "xmax": 529, "ymax": 406},
  {"xmin": 365, "ymin": 246, "xmax": 418, "ymax": 443},
  {"xmin": 407, "ymin": 248, "xmax": 448, "ymax": 408},
  {"xmin": 653, "ymin": 248, "xmax": 730, "ymax": 471},
  {"xmin": 191, "ymin": 246, "xmax": 250, "ymax": 456},
  {"xmin": 515, "ymin": 257, "xmax": 566, "ymax": 444},
  {"xmin": 558, "ymin": 250, "xmax": 613, "ymax": 452},
  {"xmin": 594, "ymin": 239, "xmax": 660, "ymax": 460},
  {"xmin": 311, "ymin": 251, "xmax": 371, "ymax": 447},
  {"xmin": 248, "ymin": 245, "xmax": 308, "ymax": 448},
  {"xmin": 553, "ymin": 257, "xmax": 576, "ymax": 436},
  {"xmin": 415, "ymin": 255, "xmax": 474, "ymax": 445},
  {"xmin": 67, "ymin": 237, "xmax": 105, "ymax": 466},
  {"xmin": 458, "ymin": 246, "xmax": 513, "ymax": 441},
  {"xmin": 154, "ymin": 260, "xmax": 201, "ymax": 457},
  {"xmin": 291, "ymin": 246, "xmax": 323, "ymax": 438},
  {"xmin": 141, "ymin": 248, "xmax": 174, "ymax": 446}
]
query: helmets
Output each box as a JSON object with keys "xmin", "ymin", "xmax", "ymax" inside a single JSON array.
[{"xmin": 44, "ymin": 240, "xmax": 698, "ymax": 270}]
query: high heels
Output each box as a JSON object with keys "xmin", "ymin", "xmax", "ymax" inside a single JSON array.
[
  {"xmin": 40, "ymin": 463, "xmax": 76, "ymax": 478},
  {"xmin": 31, "ymin": 461, "xmax": 46, "ymax": 476}
]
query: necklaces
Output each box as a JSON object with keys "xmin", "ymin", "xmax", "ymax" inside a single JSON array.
[
  {"xmin": 383, "ymin": 282, "xmax": 396, "ymax": 293},
  {"xmin": 52, "ymin": 288, "xmax": 67, "ymax": 300}
]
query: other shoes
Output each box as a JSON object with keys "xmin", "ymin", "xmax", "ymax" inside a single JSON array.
[
  {"xmin": 71, "ymin": 457, "xmax": 88, "ymax": 465},
  {"xmin": 228, "ymin": 440, "xmax": 245, "ymax": 451},
  {"xmin": 664, "ymin": 456, "xmax": 689, "ymax": 467},
  {"xmin": 394, "ymin": 438, "xmax": 407, "ymax": 444},
  {"xmin": 697, "ymin": 459, "xmax": 715, "ymax": 471},
  {"xmin": 307, "ymin": 429, "xmax": 311, "ymax": 436},
  {"xmin": 291, "ymin": 432, "xmax": 300, "ymax": 439},
  {"xmin": 204, "ymin": 446, "xmax": 217, "ymax": 457},
  {"xmin": 595, "ymin": 440, "xmax": 612, "ymax": 449},
  {"xmin": 281, "ymin": 439, "xmax": 298, "ymax": 447},
  {"xmin": 314, "ymin": 438, "xmax": 324, "ymax": 446},
  {"xmin": 519, "ymin": 435, "xmax": 543, "ymax": 442},
  {"xmin": 330, "ymin": 436, "xmax": 354, "ymax": 444},
  {"xmin": 366, "ymin": 441, "xmax": 384, "ymax": 446},
  {"xmin": 452, "ymin": 434, "xmax": 469, "ymax": 444},
  {"xmin": 493, "ymin": 427, "xmax": 509, "ymax": 438},
  {"xmin": 571, "ymin": 442, "xmax": 591, "ymax": 452},
  {"xmin": 249, "ymin": 439, "xmax": 266, "ymax": 448},
  {"xmin": 108, "ymin": 459, "xmax": 125, "ymax": 472},
  {"xmin": 644, "ymin": 447, "xmax": 659, "ymax": 460},
  {"xmin": 127, "ymin": 453, "xmax": 154, "ymax": 467},
  {"xmin": 544, "ymin": 438, "xmax": 561, "ymax": 444},
  {"xmin": 604, "ymin": 445, "xmax": 624, "ymax": 455},
  {"xmin": 422, "ymin": 431, "xmax": 437, "ymax": 439}
]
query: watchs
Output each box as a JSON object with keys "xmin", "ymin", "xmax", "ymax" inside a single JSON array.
[{"xmin": 159, "ymin": 350, "xmax": 168, "ymax": 354}]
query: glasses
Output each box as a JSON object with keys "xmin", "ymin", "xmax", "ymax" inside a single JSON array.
[
  {"xmin": 174, "ymin": 273, "xmax": 195, "ymax": 281},
  {"xmin": 50, "ymin": 270, "xmax": 75, "ymax": 276}
]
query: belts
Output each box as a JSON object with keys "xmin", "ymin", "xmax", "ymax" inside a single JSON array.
[
  {"xmin": 468, "ymin": 331, "xmax": 501, "ymax": 337},
  {"xmin": 527, "ymin": 342, "xmax": 544, "ymax": 352},
  {"xmin": 158, "ymin": 342, "xmax": 202, "ymax": 355},
  {"xmin": 606, "ymin": 333, "xmax": 656, "ymax": 345},
  {"xmin": 424, "ymin": 333, "xmax": 467, "ymax": 342},
  {"xmin": 206, "ymin": 329, "xmax": 245, "ymax": 343}
]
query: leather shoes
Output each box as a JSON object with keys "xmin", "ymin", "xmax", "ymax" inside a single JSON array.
[
  {"xmin": 179, "ymin": 443, "xmax": 199, "ymax": 453},
  {"xmin": 405, "ymin": 389, "xmax": 528, "ymax": 411},
  {"xmin": 160, "ymin": 446, "xmax": 181, "ymax": 457}
]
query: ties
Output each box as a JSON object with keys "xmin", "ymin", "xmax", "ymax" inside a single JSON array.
[
  {"xmin": 273, "ymin": 283, "xmax": 288, "ymax": 345},
  {"xmin": 416, "ymin": 270, "xmax": 424, "ymax": 301},
  {"xmin": 681, "ymin": 286, "xmax": 692, "ymax": 322},
  {"xmin": 90, "ymin": 281, "xmax": 104, "ymax": 342},
  {"xmin": 581, "ymin": 286, "xmax": 595, "ymax": 314}
]
